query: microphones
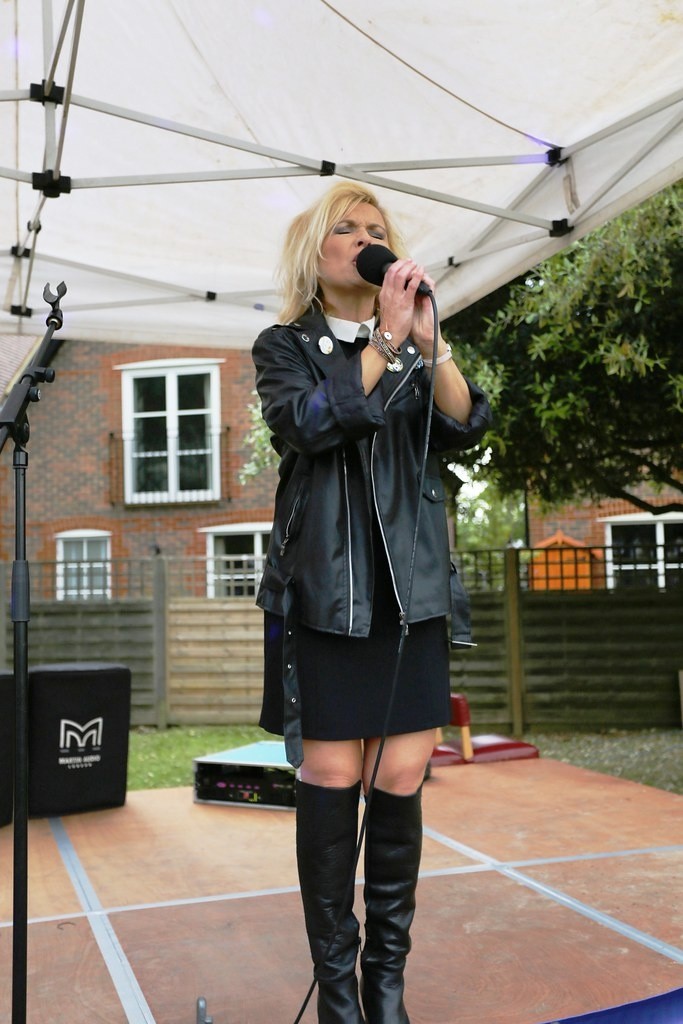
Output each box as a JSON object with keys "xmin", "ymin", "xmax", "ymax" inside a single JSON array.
[{"xmin": 356, "ymin": 244, "xmax": 433, "ymax": 296}]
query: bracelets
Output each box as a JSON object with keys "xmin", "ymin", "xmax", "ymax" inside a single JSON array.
[
  {"xmin": 369, "ymin": 327, "xmax": 402, "ymax": 364},
  {"xmin": 423, "ymin": 343, "xmax": 452, "ymax": 367}
]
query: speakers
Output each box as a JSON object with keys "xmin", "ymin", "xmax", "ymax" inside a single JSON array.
[{"xmin": 0, "ymin": 663, "xmax": 132, "ymax": 825}]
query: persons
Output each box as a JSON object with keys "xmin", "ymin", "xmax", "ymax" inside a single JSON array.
[{"xmin": 251, "ymin": 182, "xmax": 491, "ymax": 1024}]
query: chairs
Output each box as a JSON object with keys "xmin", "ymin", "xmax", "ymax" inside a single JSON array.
[{"xmin": 432, "ymin": 693, "xmax": 540, "ymax": 769}]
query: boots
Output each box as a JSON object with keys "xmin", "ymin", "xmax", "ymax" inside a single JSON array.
[
  {"xmin": 295, "ymin": 779, "xmax": 364, "ymax": 1024},
  {"xmin": 360, "ymin": 780, "xmax": 424, "ymax": 1024}
]
